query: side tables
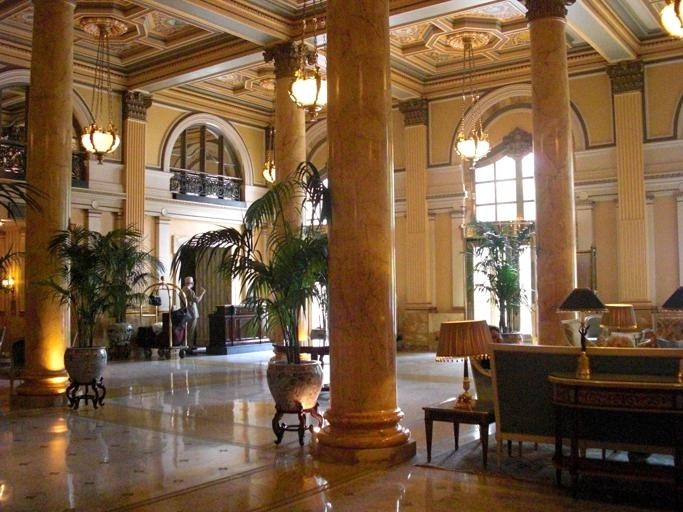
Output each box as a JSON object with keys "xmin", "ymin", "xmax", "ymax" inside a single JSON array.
[{"xmin": 422, "ymin": 399, "xmax": 495, "ymax": 466}]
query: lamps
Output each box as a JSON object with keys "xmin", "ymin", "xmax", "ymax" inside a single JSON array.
[
  {"xmin": 1, "ymin": 279, "xmax": 14, "ymax": 293},
  {"xmin": 454, "ymin": 36, "xmax": 491, "ymax": 168},
  {"xmin": 288, "ymin": 0, "xmax": 328, "ymax": 122},
  {"xmin": 170, "ymin": 162, "xmax": 333, "ymax": 413},
  {"xmin": 556, "ymin": 288, "xmax": 609, "ymax": 380},
  {"xmin": 435, "ymin": 320, "xmax": 494, "ymax": 409},
  {"xmin": 79, "ymin": 30, "xmax": 120, "ymax": 165},
  {"xmin": 102, "ymin": 224, "xmax": 165, "ymax": 345},
  {"xmin": 263, "ymin": 127, "xmax": 277, "ymax": 182},
  {"xmin": 659, "ymin": 0, "xmax": 683, "ymax": 40},
  {"xmin": 661, "ymin": 287, "xmax": 683, "ymax": 311}
]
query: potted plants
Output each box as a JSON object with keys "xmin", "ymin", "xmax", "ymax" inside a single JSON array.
[
  {"xmin": 28, "ymin": 220, "xmax": 166, "ymax": 382},
  {"xmin": 458, "ymin": 221, "xmax": 536, "ymax": 342}
]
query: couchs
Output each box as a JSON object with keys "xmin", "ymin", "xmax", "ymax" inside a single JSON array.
[{"xmin": 469, "ymin": 308, "xmax": 683, "ymax": 468}]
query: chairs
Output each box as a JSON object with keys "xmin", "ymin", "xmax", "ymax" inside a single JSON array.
[
  {"xmin": 0, "ymin": 337, "xmax": 26, "ymax": 390},
  {"xmin": 0, "ymin": 326, "xmax": 6, "ymax": 349}
]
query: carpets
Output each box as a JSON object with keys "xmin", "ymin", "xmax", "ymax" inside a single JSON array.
[{"xmin": 413, "ymin": 432, "xmax": 680, "ymax": 495}]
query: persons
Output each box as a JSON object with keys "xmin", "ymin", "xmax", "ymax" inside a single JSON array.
[{"xmin": 179, "ymin": 277, "xmax": 204, "ymax": 354}]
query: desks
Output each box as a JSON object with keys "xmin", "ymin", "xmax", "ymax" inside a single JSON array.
[
  {"xmin": 272, "ymin": 338, "xmax": 331, "ymax": 392},
  {"xmin": 547, "ymin": 372, "xmax": 683, "ymax": 488}
]
